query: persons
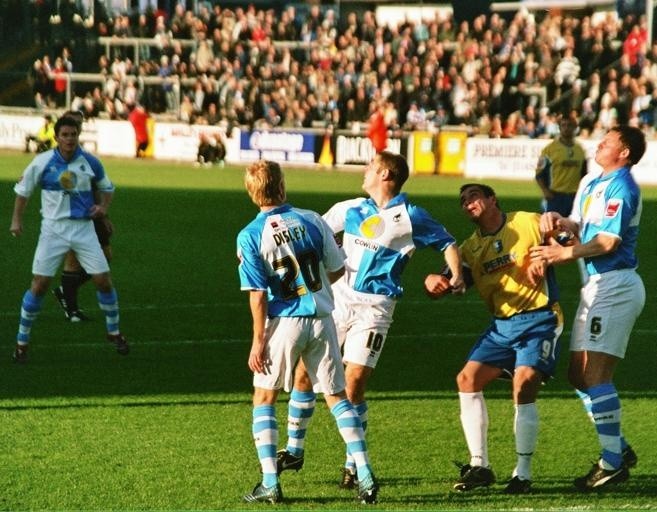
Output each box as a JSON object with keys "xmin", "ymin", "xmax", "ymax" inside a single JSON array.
[
  {"xmin": 259, "ymin": 150, "xmax": 466, "ymax": 488},
  {"xmin": 233, "ymin": 160, "xmax": 381, "ymax": 505},
  {"xmin": 8, "ymin": 116, "xmax": 130, "ymax": 363},
  {"xmin": 528, "ymin": 124, "xmax": 647, "ymax": 492},
  {"xmin": 534, "ymin": 117, "xmax": 591, "ymax": 217},
  {"xmin": 422, "ymin": 183, "xmax": 582, "ymax": 499},
  {"xmin": 50, "ymin": 110, "xmax": 115, "ymax": 324},
  {"xmin": 23, "ymin": 1, "xmax": 657, "ymax": 169}
]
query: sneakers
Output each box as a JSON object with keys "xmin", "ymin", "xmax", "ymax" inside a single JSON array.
[
  {"xmin": 50, "ymin": 285, "xmax": 72, "ymax": 311},
  {"xmin": 258, "ymin": 448, "xmax": 305, "ymax": 476},
  {"xmin": 106, "ymin": 332, "xmax": 130, "ymax": 356},
  {"xmin": 11, "ymin": 343, "xmax": 29, "ymax": 365},
  {"xmin": 64, "ymin": 309, "xmax": 92, "ymax": 323},
  {"xmin": 573, "ymin": 457, "xmax": 631, "ymax": 492},
  {"xmin": 241, "ymin": 481, "xmax": 285, "ymax": 504},
  {"xmin": 589, "ymin": 444, "xmax": 638, "ymax": 475},
  {"xmin": 498, "ymin": 475, "xmax": 533, "ymax": 495},
  {"xmin": 354, "ymin": 478, "xmax": 379, "ymax": 505},
  {"xmin": 453, "ymin": 464, "xmax": 496, "ymax": 492},
  {"xmin": 339, "ymin": 468, "xmax": 358, "ymax": 490}
]
